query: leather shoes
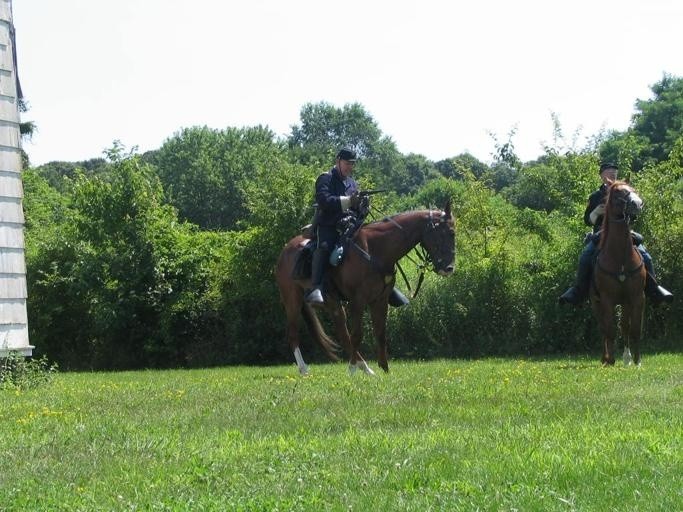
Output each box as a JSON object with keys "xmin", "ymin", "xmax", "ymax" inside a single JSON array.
[{"xmin": 304, "ymin": 287, "xmax": 326, "ymax": 304}]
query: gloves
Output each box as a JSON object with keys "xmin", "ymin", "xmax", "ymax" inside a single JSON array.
[
  {"xmin": 590, "ymin": 204, "xmax": 606, "ymax": 225},
  {"xmin": 340, "ymin": 193, "xmax": 370, "ymax": 212}
]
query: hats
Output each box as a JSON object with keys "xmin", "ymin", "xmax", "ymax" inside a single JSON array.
[
  {"xmin": 337, "ymin": 148, "xmax": 357, "ymax": 163},
  {"xmin": 599, "ymin": 163, "xmax": 619, "ymax": 174}
]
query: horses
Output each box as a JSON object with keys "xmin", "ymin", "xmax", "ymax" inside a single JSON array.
[
  {"xmin": 589, "ymin": 171, "xmax": 647, "ymax": 369},
  {"xmin": 275, "ymin": 199, "xmax": 456, "ymax": 378}
]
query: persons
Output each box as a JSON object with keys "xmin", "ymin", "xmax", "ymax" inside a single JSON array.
[
  {"xmin": 303, "ymin": 149, "xmax": 372, "ymax": 305},
  {"xmin": 560, "ymin": 161, "xmax": 656, "ymax": 304}
]
